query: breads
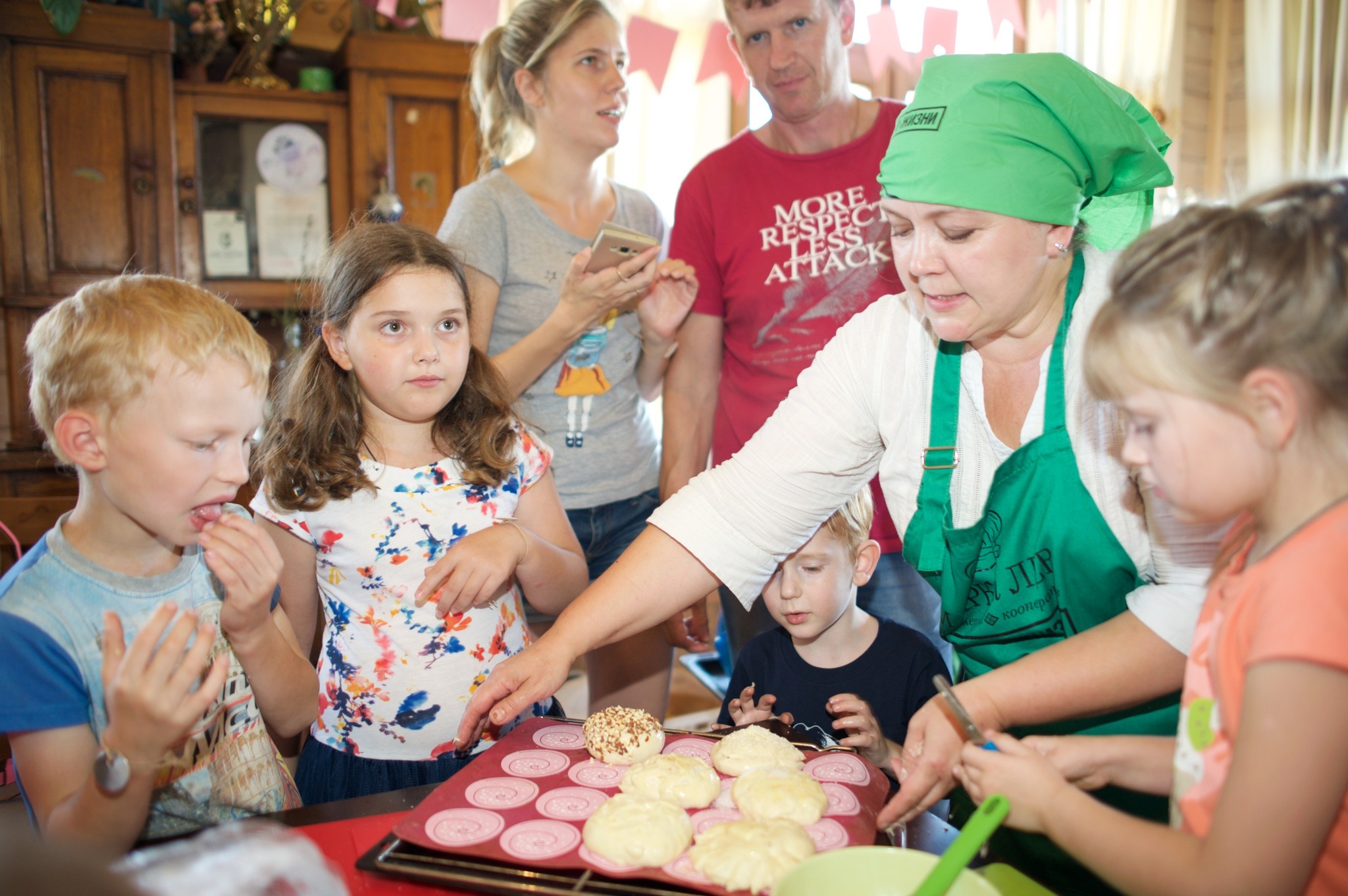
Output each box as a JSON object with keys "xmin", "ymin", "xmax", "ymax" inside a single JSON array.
[
  {"xmin": 618, "ymin": 753, "xmax": 721, "ymax": 809},
  {"xmin": 582, "ymin": 793, "xmax": 693, "ymax": 868},
  {"xmin": 583, "ymin": 705, "xmax": 666, "ymax": 765},
  {"xmin": 689, "ymin": 817, "xmax": 819, "ymax": 895},
  {"xmin": 731, "ymin": 767, "xmax": 829, "ymax": 826},
  {"xmin": 711, "ymin": 725, "xmax": 807, "ymax": 776}
]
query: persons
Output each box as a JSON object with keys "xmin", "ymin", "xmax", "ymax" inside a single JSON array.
[
  {"xmin": 953, "ymin": 173, "xmax": 1348, "ymax": 896},
  {"xmin": 251, "ymin": 220, "xmax": 586, "ymax": 794},
  {"xmin": 717, "ymin": 481, "xmax": 954, "ymax": 814},
  {"xmin": 451, "ymin": 53, "xmax": 1184, "ymax": 896},
  {"xmin": 423, "ymin": 0, "xmax": 700, "ymax": 728},
  {"xmin": 0, "ymin": 275, "xmax": 320, "ymax": 861},
  {"xmin": 659, "ymin": 0, "xmax": 941, "ymax": 682}
]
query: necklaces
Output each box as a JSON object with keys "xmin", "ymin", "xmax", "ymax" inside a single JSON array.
[{"xmin": 362, "ymin": 439, "xmax": 377, "ymax": 461}]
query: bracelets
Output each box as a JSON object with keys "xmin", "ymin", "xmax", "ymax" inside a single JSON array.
[
  {"xmin": 93, "ymin": 726, "xmax": 170, "ymax": 793},
  {"xmin": 640, "ymin": 335, "xmax": 678, "ymax": 358}
]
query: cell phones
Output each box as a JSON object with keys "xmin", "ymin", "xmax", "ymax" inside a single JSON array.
[
  {"xmin": 584, "ymin": 228, "xmax": 657, "ymax": 276},
  {"xmin": 932, "ymin": 673, "xmax": 998, "ymax": 752}
]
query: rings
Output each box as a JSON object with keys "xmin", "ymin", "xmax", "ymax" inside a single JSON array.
[
  {"xmin": 635, "ymin": 289, "xmax": 639, "ymax": 295},
  {"xmin": 615, "ymin": 266, "xmax": 628, "ymax": 281}
]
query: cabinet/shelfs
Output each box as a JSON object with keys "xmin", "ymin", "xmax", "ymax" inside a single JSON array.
[{"xmin": 0, "ymin": 8, "xmax": 489, "ymax": 544}]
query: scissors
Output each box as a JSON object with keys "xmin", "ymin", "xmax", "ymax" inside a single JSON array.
[{"xmin": 932, "ymin": 674, "xmax": 999, "ymax": 752}]
